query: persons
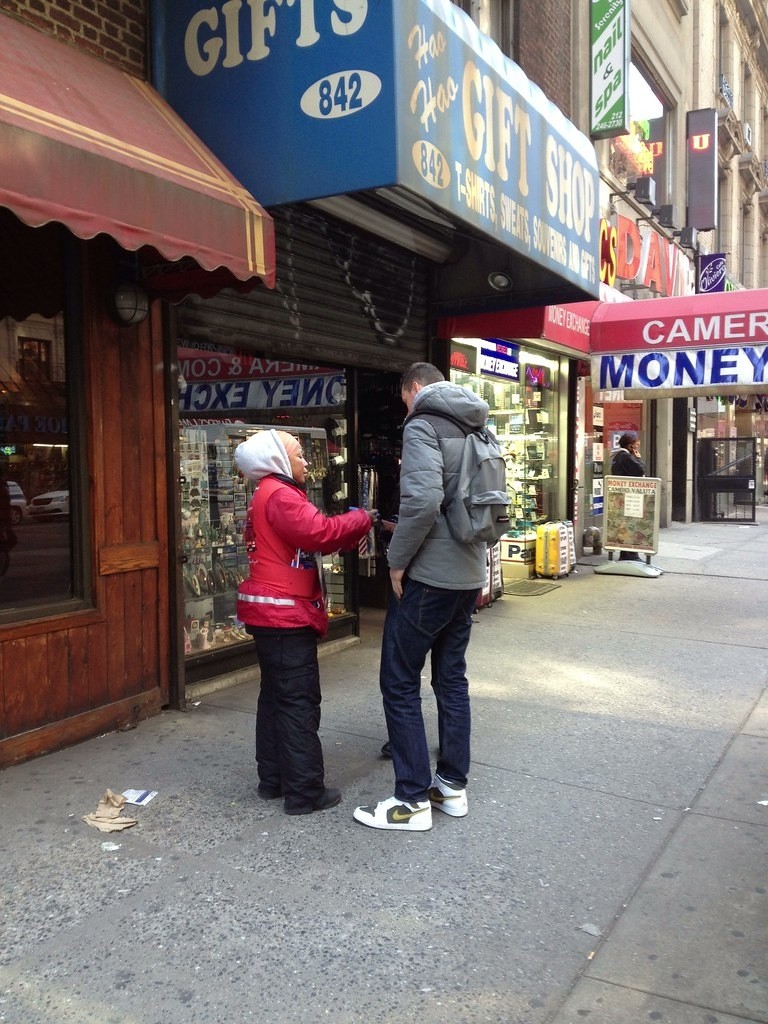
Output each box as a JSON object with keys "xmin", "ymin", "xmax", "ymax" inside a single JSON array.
[
  {"xmin": 234, "ymin": 429, "xmax": 379, "ymax": 816},
  {"xmin": 611, "ymin": 433, "xmax": 644, "ymax": 476},
  {"xmin": 0, "ymin": 445, "xmax": 18, "ymax": 576},
  {"xmin": 632, "ymin": 434, "xmax": 640, "ymax": 461},
  {"xmin": 353, "ymin": 361, "xmax": 510, "ymax": 831},
  {"xmin": 368, "ymin": 465, "xmax": 400, "ymax": 759}
]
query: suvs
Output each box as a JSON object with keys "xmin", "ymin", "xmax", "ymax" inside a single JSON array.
[{"xmin": 6, "ymin": 480, "xmax": 29, "ymax": 526}]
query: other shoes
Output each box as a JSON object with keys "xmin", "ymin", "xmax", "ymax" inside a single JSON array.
[{"xmin": 380, "ymin": 741, "xmax": 393, "ymax": 756}]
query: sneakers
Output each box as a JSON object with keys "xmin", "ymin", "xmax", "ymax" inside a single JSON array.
[
  {"xmin": 425, "ymin": 773, "xmax": 469, "ymax": 817},
  {"xmin": 353, "ymin": 795, "xmax": 433, "ymax": 831}
]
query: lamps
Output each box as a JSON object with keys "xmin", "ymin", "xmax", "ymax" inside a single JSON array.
[
  {"xmin": 607, "ymin": 175, "xmax": 658, "ymax": 213},
  {"xmin": 671, "ymin": 225, "xmax": 699, "ymax": 250},
  {"xmin": 635, "ymin": 204, "xmax": 681, "ymax": 231}
]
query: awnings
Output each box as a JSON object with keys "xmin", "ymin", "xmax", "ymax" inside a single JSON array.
[
  {"xmin": 145, "ymin": 0, "xmax": 601, "ymax": 312},
  {"xmin": 0, "ymin": 13, "xmax": 275, "ymax": 303}
]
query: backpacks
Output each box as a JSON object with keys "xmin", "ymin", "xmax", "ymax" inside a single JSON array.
[{"xmin": 403, "ymin": 407, "xmax": 513, "ymax": 548}]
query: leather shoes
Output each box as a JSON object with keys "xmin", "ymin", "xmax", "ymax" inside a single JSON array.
[
  {"xmin": 284, "ymin": 788, "xmax": 342, "ymax": 814},
  {"xmin": 257, "ymin": 785, "xmax": 285, "ymax": 799}
]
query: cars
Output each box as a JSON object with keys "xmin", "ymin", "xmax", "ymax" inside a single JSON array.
[{"xmin": 26, "ymin": 480, "xmax": 70, "ymax": 522}]
